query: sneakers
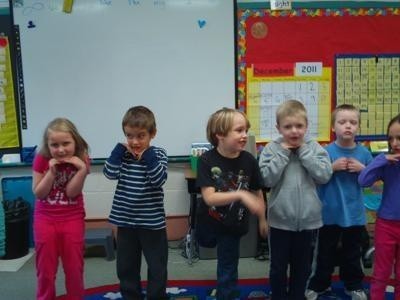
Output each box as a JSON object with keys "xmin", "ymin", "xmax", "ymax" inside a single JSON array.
[
  {"xmin": 304, "ymin": 285, "xmax": 331, "ymax": 300},
  {"xmin": 343, "ymin": 285, "xmax": 367, "ymax": 300}
]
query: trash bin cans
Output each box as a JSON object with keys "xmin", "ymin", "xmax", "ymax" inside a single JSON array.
[{"xmin": 0, "ymin": 198, "xmax": 31, "ymax": 259}]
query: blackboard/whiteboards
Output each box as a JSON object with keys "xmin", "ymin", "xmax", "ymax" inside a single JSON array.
[{"xmin": 9, "ymin": 0, "xmax": 238, "ymax": 163}]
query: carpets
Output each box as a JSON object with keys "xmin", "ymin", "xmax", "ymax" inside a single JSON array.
[{"xmin": 49, "ymin": 273, "xmax": 400, "ymax": 300}]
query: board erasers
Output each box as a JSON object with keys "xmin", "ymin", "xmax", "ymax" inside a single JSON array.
[{"xmin": 62, "ymin": 0, "xmax": 73, "ymax": 13}]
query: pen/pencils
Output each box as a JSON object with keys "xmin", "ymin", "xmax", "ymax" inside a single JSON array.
[{"xmin": 24, "ymin": 146, "xmax": 37, "ymax": 161}]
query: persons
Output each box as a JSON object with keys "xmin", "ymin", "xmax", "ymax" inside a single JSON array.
[
  {"xmin": 31, "ymin": 117, "xmax": 91, "ymax": 300},
  {"xmin": 195, "ymin": 106, "xmax": 268, "ymax": 300},
  {"xmin": 304, "ymin": 104, "xmax": 374, "ymax": 300},
  {"xmin": 103, "ymin": 105, "xmax": 169, "ymax": 300},
  {"xmin": 259, "ymin": 99, "xmax": 333, "ymax": 300},
  {"xmin": 358, "ymin": 112, "xmax": 400, "ymax": 300}
]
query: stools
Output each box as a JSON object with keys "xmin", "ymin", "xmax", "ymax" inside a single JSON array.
[{"xmin": 84, "ymin": 228, "xmax": 117, "ymax": 261}]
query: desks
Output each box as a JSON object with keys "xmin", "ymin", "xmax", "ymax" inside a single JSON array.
[
  {"xmin": 0, "ymin": 246, "xmax": 37, "ymax": 300},
  {"xmin": 185, "ymin": 161, "xmax": 259, "ymax": 259}
]
